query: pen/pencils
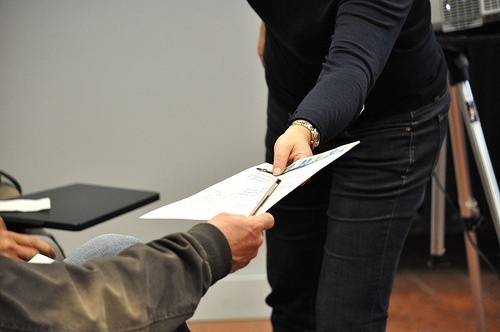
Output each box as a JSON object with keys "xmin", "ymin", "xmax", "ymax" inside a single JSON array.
[{"xmin": 247, "ymin": 179, "xmax": 281, "ymax": 215}]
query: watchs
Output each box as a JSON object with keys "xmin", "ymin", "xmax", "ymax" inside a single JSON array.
[{"xmin": 290, "ymin": 119, "xmax": 322, "ymax": 151}]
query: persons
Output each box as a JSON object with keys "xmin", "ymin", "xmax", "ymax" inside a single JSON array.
[
  {"xmin": 247, "ymin": 0, "xmax": 454, "ymax": 332},
  {"xmin": 0, "ymin": 210, "xmax": 275, "ymax": 331}
]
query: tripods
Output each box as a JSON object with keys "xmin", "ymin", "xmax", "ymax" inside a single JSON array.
[{"xmin": 429, "ymin": 38, "xmax": 500, "ymax": 331}]
